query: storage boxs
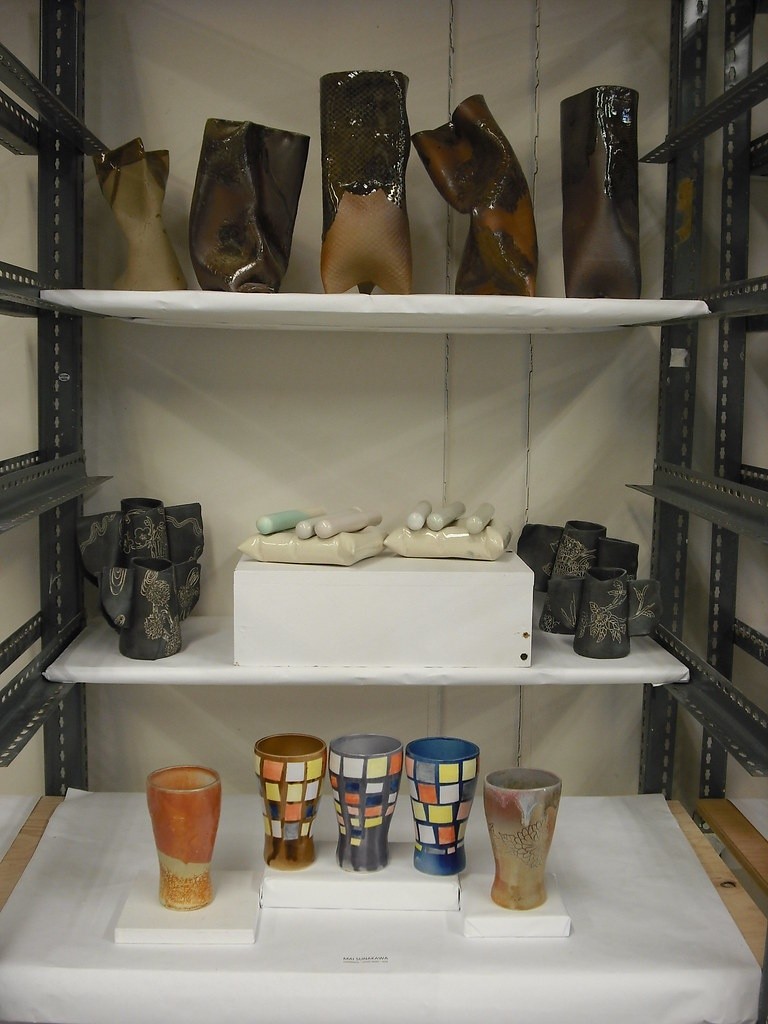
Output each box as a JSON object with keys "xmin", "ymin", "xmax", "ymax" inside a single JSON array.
[{"xmin": 235, "ymin": 547, "xmax": 534, "ymax": 669}]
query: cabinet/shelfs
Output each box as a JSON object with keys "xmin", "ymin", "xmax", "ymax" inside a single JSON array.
[{"xmin": 0, "ymin": 0, "xmax": 768, "ymax": 1024}]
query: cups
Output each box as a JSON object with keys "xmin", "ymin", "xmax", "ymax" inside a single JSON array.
[
  {"xmin": 146, "ymin": 765, "xmax": 225, "ymax": 909},
  {"xmin": 403, "ymin": 738, "xmax": 481, "ymax": 874},
  {"xmin": 252, "ymin": 733, "xmax": 326, "ymax": 871},
  {"xmin": 484, "ymin": 768, "xmax": 564, "ymax": 909},
  {"xmin": 327, "ymin": 735, "xmax": 404, "ymax": 871}
]
